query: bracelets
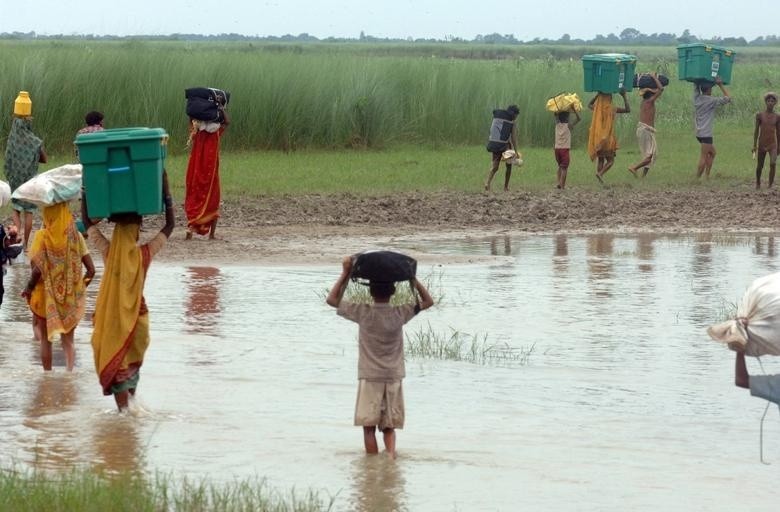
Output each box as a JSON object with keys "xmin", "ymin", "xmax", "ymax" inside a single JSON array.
[{"xmin": 167, "ymin": 207, "xmax": 172, "ymax": 209}]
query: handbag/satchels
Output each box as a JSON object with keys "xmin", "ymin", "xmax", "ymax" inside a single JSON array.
[
  {"xmin": 184, "ymin": 85, "xmax": 229, "ymax": 124},
  {"xmin": 346, "ymin": 252, "xmax": 416, "ymax": 281}
]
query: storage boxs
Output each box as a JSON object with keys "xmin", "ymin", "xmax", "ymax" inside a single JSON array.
[
  {"xmin": 72, "ymin": 125, "xmax": 169, "ymax": 219},
  {"xmin": 674, "ymin": 40, "xmax": 738, "ymax": 88},
  {"xmin": 579, "ymin": 51, "xmax": 641, "ymax": 94}
]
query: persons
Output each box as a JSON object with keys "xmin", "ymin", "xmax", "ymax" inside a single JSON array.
[
  {"xmin": 751, "ymin": 92, "xmax": 780, "ymax": 186},
  {"xmin": 692, "ymin": 82, "xmax": 731, "ymax": 181},
  {"xmin": 484, "ymin": 105, "xmax": 521, "ymax": 191},
  {"xmin": 21, "ymin": 201, "xmax": 95, "ymax": 371},
  {"xmin": 628, "ymin": 74, "xmax": 664, "ymax": 179},
  {"xmin": 325, "ymin": 257, "xmax": 434, "ymax": 455},
  {"xmin": 0, "ymin": 181, "xmax": 23, "ymax": 297},
  {"xmin": 735, "ymin": 351, "xmax": 780, "ymax": 411},
  {"xmin": 1, "ymin": 115, "xmax": 48, "ymax": 248},
  {"xmin": 184, "ymin": 104, "xmax": 231, "ymax": 239},
  {"xmin": 74, "ymin": 111, "xmax": 105, "ymax": 163},
  {"xmin": 82, "ymin": 168, "xmax": 174, "ymax": 412},
  {"xmin": 587, "ymin": 92, "xmax": 630, "ymax": 183},
  {"xmin": 553, "ymin": 103, "xmax": 581, "ymax": 191}
]
